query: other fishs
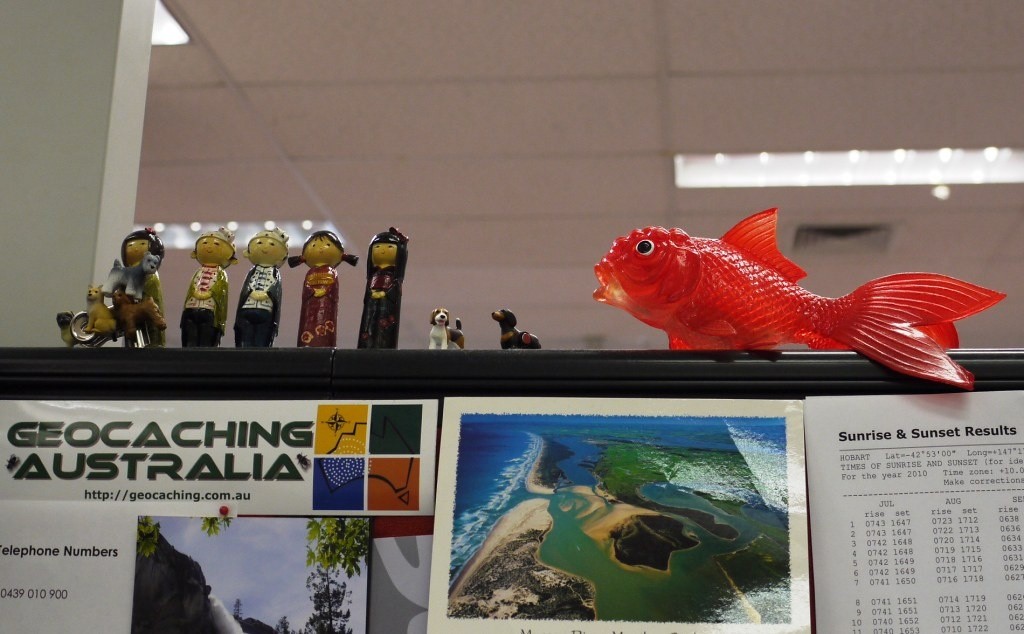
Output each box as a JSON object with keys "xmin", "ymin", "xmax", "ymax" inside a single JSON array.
[{"xmin": 593, "ymin": 207, "xmax": 1008, "ymax": 391}]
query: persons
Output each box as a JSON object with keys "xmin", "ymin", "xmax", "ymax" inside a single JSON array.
[
  {"xmin": 233, "ymin": 227, "xmax": 289, "ymax": 348},
  {"xmin": 179, "ymin": 226, "xmax": 237, "ymax": 348},
  {"xmin": 288, "ymin": 231, "xmax": 359, "ymax": 347},
  {"xmin": 121, "ymin": 228, "xmax": 167, "ymax": 347},
  {"xmin": 357, "ymin": 226, "xmax": 409, "ymax": 349}
]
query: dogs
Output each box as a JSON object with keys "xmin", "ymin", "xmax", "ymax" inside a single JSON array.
[
  {"xmin": 492, "ymin": 310, "xmax": 541, "ymax": 349},
  {"xmin": 428, "ymin": 308, "xmax": 466, "ymax": 351}
]
